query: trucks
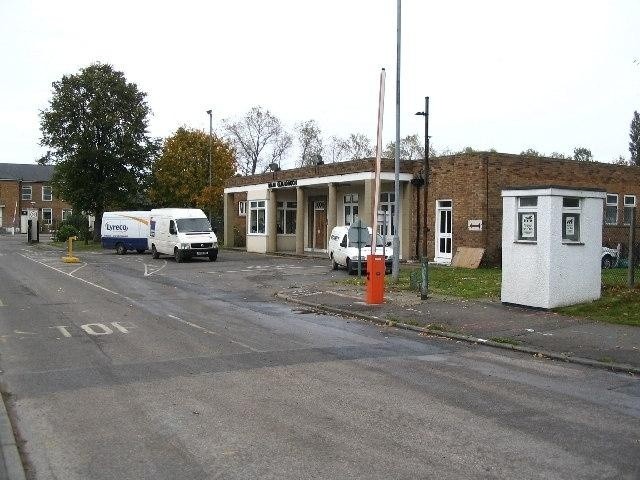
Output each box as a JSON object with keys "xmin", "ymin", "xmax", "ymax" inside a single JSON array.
[
  {"xmin": 327, "ymin": 224, "xmax": 393, "ymax": 276},
  {"xmin": 147, "ymin": 208, "xmax": 219, "ymax": 262},
  {"xmin": 100, "ymin": 210, "xmax": 151, "ymax": 255}
]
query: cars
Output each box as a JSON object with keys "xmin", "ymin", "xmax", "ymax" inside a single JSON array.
[{"xmin": 601, "ymin": 245, "xmax": 619, "ymax": 269}]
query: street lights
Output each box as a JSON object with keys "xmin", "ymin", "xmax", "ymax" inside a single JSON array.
[
  {"xmin": 413, "ymin": 96, "xmax": 430, "ymax": 300},
  {"xmin": 206, "ymin": 109, "xmax": 215, "ymax": 226}
]
